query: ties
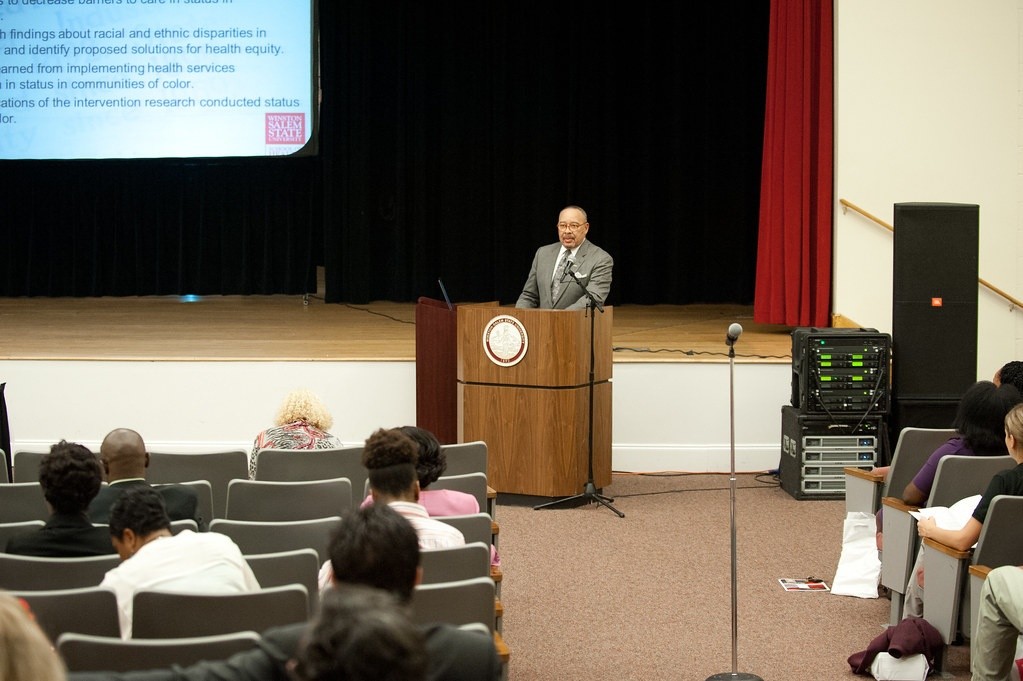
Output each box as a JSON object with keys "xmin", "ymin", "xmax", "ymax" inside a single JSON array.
[{"xmin": 551, "ymin": 248, "xmax": 572, "ymax": 306}]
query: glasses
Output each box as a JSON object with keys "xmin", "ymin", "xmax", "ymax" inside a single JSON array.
[{"xmin": 557, "ymin": 222, "xmax": 588, "ymax": 232}]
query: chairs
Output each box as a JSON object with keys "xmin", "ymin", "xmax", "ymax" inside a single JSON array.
[
  {"xmin": 961, "ymin": 494, "xmax": 1023, "ymax": 639},
  {"xmin": 0, "ymin": 441, "xmax": 509, "ymax": 671},
  {"xmin": 914, "ymin": 455, "xmax": 1018, "ymax": 565},
  {"xmin": 879, "ymin": 428, "xmax": 960, "ymax": 507}
]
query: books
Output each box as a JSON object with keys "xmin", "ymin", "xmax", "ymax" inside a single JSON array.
[{"xmin": 907, "ymin": 495, "xmax": 983, "ymax": 548}]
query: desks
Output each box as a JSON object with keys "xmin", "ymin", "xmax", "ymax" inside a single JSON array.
[{"xmin": 843, "ymin": 465, "xmax": 1023, "ymax": 681}]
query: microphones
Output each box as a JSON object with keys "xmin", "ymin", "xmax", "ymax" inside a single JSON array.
[
  {"xmin": 726, "ymin": 323, "xmax": 742, "ymax": 346},
  {"xmin": 560, "ymin": 255, "xmax": 577, "ymax": 282}
]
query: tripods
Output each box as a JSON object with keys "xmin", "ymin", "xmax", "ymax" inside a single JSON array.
[{"xmin": 534, "ymin": 268, "xmax": 625, "ymax": 518}]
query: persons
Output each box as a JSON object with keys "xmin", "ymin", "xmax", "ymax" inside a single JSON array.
[
  {"xmin": 97, "ymin": 485, "xmax": 261, "ymax": 638},
  {"xmin": 4, "ymin": 441, "xmax": 118, "ymax": 557},
  {"xmin": 874, "ymin": 361, "xmax": 1023, "ymax": 681},
  {"xmin": 249, "ymin": 389, "xmax": 343, "ymax": 481},
  {"xmin": 515, "ymin": 206, "xmax": 613, "ymax": 309},
  {"xmin": 318, "ymin": 426, "xmax": 501, "ymax": 598},
  {"xmin": 0, "ymin": 507, "xmax": 503, "ymax": 681},
  {"xmin": 87, "ymin": 427, "xmax": 200, "ymax": 524}
]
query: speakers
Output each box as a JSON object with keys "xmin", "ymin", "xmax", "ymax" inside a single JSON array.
[
  {"xmin": 893, "ymin": 202, "xmax": 979, "ymax": 400},
  {"xmin": 889, "ymin": 398, "xmax": 966, "ymax": 459}
]
